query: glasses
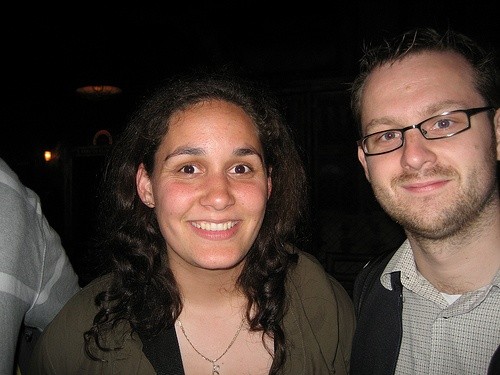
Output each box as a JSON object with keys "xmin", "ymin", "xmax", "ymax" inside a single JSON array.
[{"xmin": 356, "ymin": 105, "xmax": 497, "ymax": 156}]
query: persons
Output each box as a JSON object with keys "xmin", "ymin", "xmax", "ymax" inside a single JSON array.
[
  {"xmin": 350, "ymin": 24, "xmax": 500, "ymax": 375},
  {"xmin": 22, "ymin": 82, "xmax": 358, "ymax": 375},
  {"xmin": 0, "ymin": 159, "xmax": 82, "ymax": 375}
]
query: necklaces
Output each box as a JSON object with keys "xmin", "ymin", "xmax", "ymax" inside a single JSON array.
[{"xmin": 176, "ymin": 311, "xmax": 246, "ymax": 375}]
